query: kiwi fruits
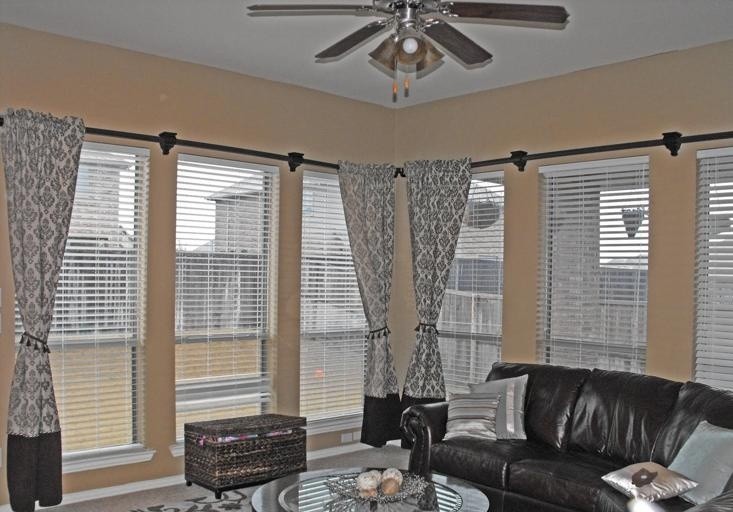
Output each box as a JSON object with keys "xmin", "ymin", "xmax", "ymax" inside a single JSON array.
[{"xmin": 382, "ymin": 479, "xmax": 399, "ymax": 495}]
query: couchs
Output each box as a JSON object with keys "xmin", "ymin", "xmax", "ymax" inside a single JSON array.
[{"xmin": 401, "ymin": 362, "xmax": 733, "ymax": 511}]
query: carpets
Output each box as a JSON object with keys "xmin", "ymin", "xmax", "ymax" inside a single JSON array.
[{"xmin": 125, "ymin": 485, "xmax": 257, "ymax": 511}]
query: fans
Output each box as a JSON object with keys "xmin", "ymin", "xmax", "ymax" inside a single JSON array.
[{"xmin": 246, "ymin": 1, "xmax": 569, "ymax": 66}]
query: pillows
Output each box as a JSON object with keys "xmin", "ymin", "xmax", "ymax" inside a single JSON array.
[
  {"xmin": 464, "ymin": 374, "xmax": 529, "ymax": 443},
  {"xmin": 667, "ymin": 420, "xmax": 733, "ymax": 505},
  {"xmin": 600, "ymin": 460, "xmax": 700, "ymax": 503},
  {"xmin": 445, "ymin": 392, "xmax": 499, "ymax": 442}
]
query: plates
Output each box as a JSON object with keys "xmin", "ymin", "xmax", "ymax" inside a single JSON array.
[{"xmin": 325, "ymin": 470, "xmax": 427, "ymax": 504}]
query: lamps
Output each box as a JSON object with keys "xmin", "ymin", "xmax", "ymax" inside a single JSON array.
[{"xmin": 368, "ymin": 0, "xmax": 444, "ymax": 102}]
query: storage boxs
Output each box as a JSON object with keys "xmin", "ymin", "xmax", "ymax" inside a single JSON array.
[{"xmin": 184, "ymin": 414, "xmax": 306, "ymax": 500}]
